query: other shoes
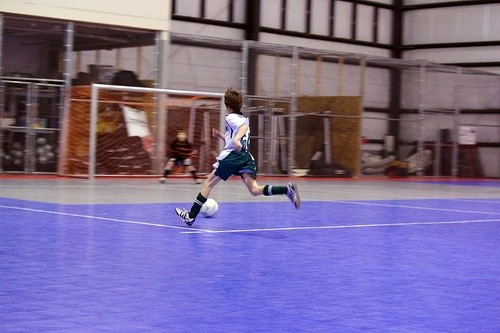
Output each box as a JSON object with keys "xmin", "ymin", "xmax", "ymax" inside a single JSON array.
[
  {"xmin": 160, "ymin": 178, "xmax": 165, "ymax": 183},
  {"xmin": 196, "ymin": 179, "xmax": 201, "ymax": 184}
]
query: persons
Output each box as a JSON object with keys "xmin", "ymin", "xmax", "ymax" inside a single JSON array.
[
  {"xmin": 174, "ymin": 86, "xmax": 301, "ymax": 227},
  {"xmin": 158, "ymin": 129, "xmax": 202, "ymax": 185}
]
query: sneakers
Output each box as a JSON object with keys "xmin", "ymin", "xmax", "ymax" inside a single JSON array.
[
  {"xmin": 175, "ymin": 207, "xmax": 195, "ymax": 226},
  {"xmin": 286, "ymin": 182, "xmax": 300, "ymax": 209}
]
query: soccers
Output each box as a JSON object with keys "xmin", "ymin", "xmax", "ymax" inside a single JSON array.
[{"xmin": 200, "ymin": 199, "xmax": 218, "ymax": 218}]
